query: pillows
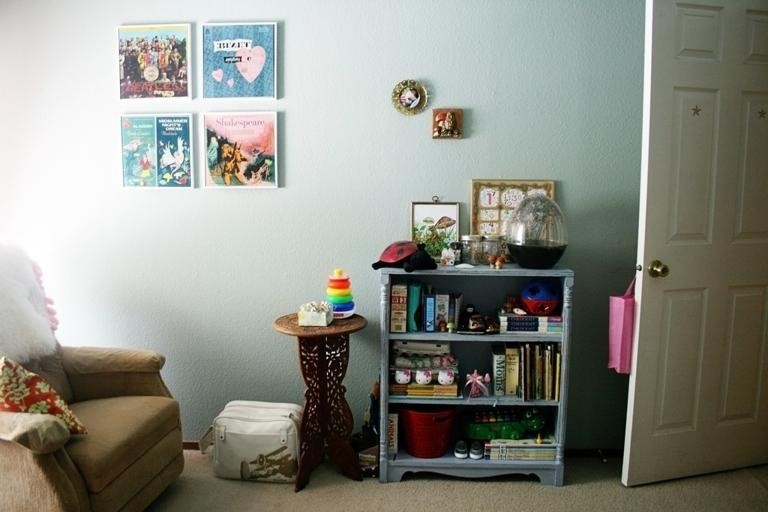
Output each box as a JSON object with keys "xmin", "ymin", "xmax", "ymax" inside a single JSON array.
[{"xmin": 1, "ymin": 356, "xmax": 88, "ymax": 435}]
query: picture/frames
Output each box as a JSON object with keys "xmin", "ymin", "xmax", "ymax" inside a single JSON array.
[
  {"xmin": 200, "ymin": 22, "xmax": 277, "ymax": 101},
  {"xmin": 409, "ymin": 195, "xmax": 461, "ymax": 263},
  {"xmin": 119, "ymin": 114, "xmax": 193, "ymax": 189},
  {"xmin": 116, "ymin": 23, "xmax": 192, "ymax": 100},
  {"xmin": 471, "ymin": 179, "xmax": 556, "ymax": 256},
  {"xmin": 391, "ymin": 79, "xmax": 428, "ymax": 116},
  {"xmin": 199, "ymin": 111, "xmax": 279, "ymax": 189}
]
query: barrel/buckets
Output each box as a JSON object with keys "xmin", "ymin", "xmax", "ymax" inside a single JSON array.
[{"xmin": 404, "ymin": 411, "xmax": 450, "ymax": 458}]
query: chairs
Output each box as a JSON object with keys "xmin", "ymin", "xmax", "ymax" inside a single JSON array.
[{"xmin": 0, "ymin": 241, "xmax": 185, "ymax": 511}]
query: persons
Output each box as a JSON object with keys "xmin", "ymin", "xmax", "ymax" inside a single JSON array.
[{"xmin": 120, "ymin": 35, "xmax": 186, "ymax": 82}]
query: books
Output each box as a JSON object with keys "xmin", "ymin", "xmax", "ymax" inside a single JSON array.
[
  {"xmin": 492, "ymin": 343, "xmax": 559, "ymax": 402},
  {"xmin": 485, "ymin": 438, "xmax": 557, "ymax": 461},
  {"xmin": 497, "ymin": 315, "xmax": 564, "ymax": 335}
]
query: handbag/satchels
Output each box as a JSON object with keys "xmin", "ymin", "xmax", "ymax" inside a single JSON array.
[
  {"xmin": 607, "ymin": 294, "xmax": 635, "ymax": 375},
  {"xmin": 211, "ymin": 400, "xmax": 304, "ymax": 483}
]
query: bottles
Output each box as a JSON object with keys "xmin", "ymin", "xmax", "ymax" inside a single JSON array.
[
  {"xmin": 482, "ymin": 234, "xmax": 503, "ymax": 266},
  {"xmin": 461, "ymin": 235, "xmax": 483, "ymax": 265}
]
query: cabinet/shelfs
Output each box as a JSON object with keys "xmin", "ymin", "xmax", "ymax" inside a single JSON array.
[
  {"xmin": 378, "ymin": 261, "xmax": 577, "ymax": 487},
  {"xmin": 271, "ymin": 313, "xmax": 367, "ymax": 494}
]
